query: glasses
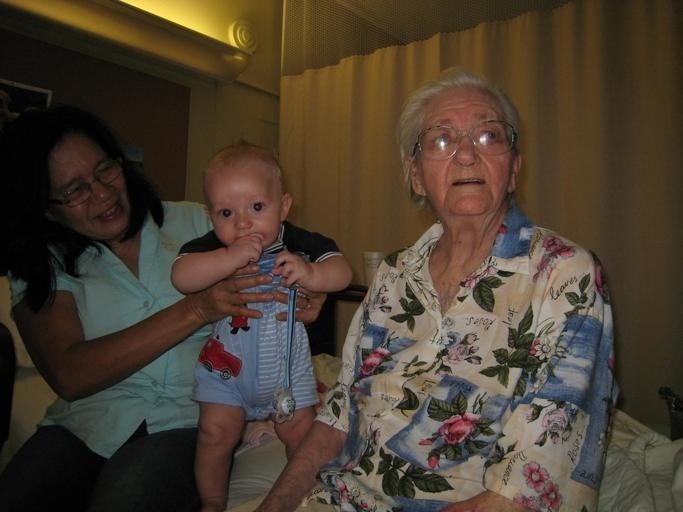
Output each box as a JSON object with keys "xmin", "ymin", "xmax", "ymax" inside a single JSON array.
[
  {"xmin": 52, "ymin": 156, "xmax": 126, "ymax": 207},
  {"xmin": 409, "ymin": 119, "xmax": 519, "ymax": 161}
]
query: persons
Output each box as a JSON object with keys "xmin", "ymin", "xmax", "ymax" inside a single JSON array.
[
  {"xmin": 0, "ymin": 100, "xmax": 327, "ymax": 511},
  {"xmin": 168, "ymin": 136, "xmax": 357, "ymax": 512},
  {"xmin": 253, "ymin": 64, "xmax": 621, "ymax": 512}
]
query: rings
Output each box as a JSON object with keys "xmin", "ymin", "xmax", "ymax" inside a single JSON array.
[{"xmin": 303, "ymin": 297, "xmax": 310, "ymax": 309}]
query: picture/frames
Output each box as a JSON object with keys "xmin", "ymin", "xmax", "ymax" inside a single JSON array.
[{"xmin": 0, "ymin": 77, "xmax": 53, "ymax": 129}]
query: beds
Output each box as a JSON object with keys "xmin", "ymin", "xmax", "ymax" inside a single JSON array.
[{"xmin": 1, "ymin": 276, "xmax": 682, "ymax": 512}]
